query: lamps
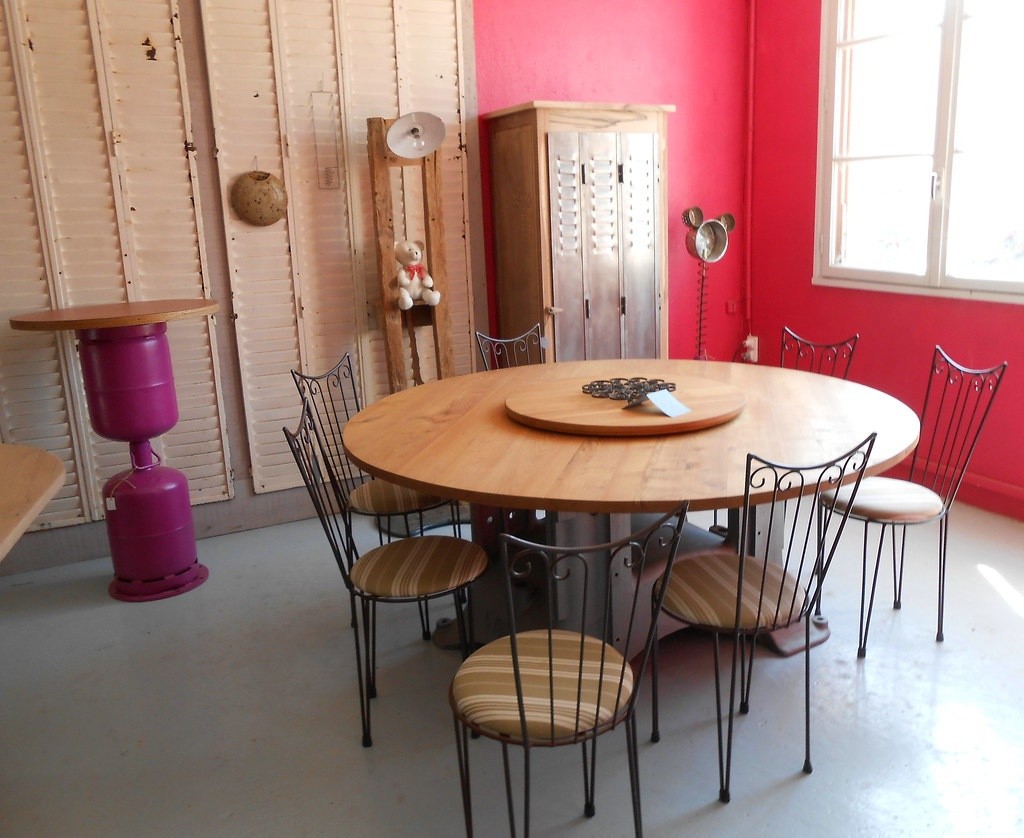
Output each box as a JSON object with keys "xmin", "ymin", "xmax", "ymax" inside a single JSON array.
[{"xmin": 386, "ymin": 111, "xmax": 446, "ymax": 158}]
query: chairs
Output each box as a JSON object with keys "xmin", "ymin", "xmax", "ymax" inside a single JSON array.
[
  {"xmin": 782, "ymin": 325, "xmax": 860, "ymax": 380},
  {"xmin": 291, "ymin": 352, "xmax": 459, "ymax": 640},
  {"xmin": 476, "ymin": 322, "xmax": 544, "ymax": 370},
  {"xmin": 813, "ymin": 346, "xmax": 1004, "ymax": 659},
  {"xmin": 451, "ymin": 502, "xmax": 690, "ymax": 837},
  {"xmin": 9, "ymin": 297, "xmax": 218, "ymax": 604},
  {"xmin": 282, "ymin": 396, "xmax": 489, "ymax": 745},
  {"xmin": 650, "ymin": 433, "xmax": 878, "ymax": 804}
]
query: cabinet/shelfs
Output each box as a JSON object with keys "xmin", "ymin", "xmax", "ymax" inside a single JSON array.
[{"xmin": 489, "ymin": 99, "xmax": 678, "ymax": 367}]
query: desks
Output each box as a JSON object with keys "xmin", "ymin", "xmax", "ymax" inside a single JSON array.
[
  {"xmin": 1, "ymin": 443, "xmax": 67, "ymax": 562},
  {"xmin": 340, "ymin": 360, "xmax": 923, "ymax": 659}
]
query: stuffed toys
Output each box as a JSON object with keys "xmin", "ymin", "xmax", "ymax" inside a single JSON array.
[{"xmin": 393, "ymin": 241, "xmax": 441, "ymax": 310}]
